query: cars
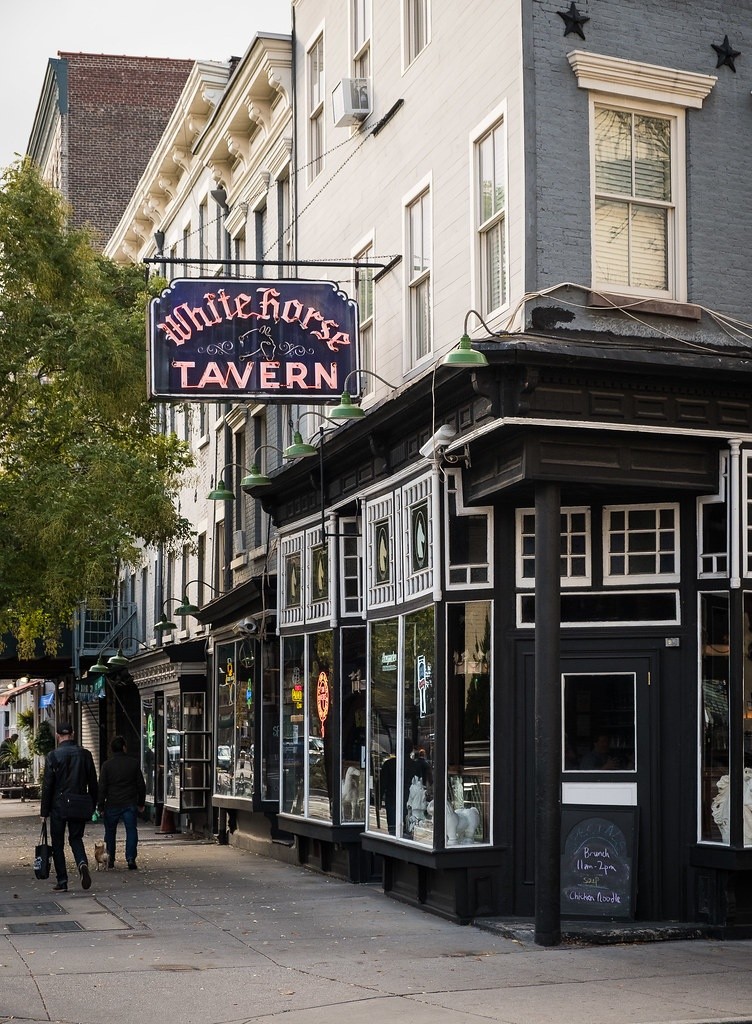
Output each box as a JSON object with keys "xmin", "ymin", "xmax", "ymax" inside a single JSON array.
[
  {"xmin": 370, "ymin": 738, "xmax": 391, "ymax": 807},
  {"xmin": 217, "ymin": 745, "xmax": 232, "ymax": 770},
  {"xmin": 309, "ymin": 736, "xmax": 325, "ymax": 764}
]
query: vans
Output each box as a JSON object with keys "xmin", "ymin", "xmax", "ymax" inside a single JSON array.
[{"xmin": 150, "ymin": 729, "xmax": 181, "ymax": 764}]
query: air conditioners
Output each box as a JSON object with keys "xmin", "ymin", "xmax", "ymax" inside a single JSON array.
[{"xmin": 331, "ymin": 77, "xmax": 369, "ymax": 127}]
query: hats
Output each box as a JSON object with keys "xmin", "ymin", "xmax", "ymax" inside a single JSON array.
[{"xmin": 57, "ymin": 723, "xmax": 73, "ymax": 735}]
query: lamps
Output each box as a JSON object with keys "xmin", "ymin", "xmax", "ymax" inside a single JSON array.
[
  {"xmin": 153, "ymin": 597, "xmax": 183, "ymax": 630},
  {"xmin": 281, "ymin": 411, "xmax": 341, "ymax": 458},
  {"xmin": 89, "ymin": 648, "xmax": 128, "ymax": 673},
  {"xmin": 206, "ymin": 463, "xmax": 252, "ymax": 500},
  {"xmin": 238, "ymin": 444, "xmax": 284, "ymax": 486},
  {"xmin": 441, "ymin": 309, "xmax": 514, "ymax": 367},
  {"xmin": 174, "ymin": 580, "xmax": 226, "ymax": 616},
  {"xmin": 106, "ymin": 637, "xmax": 150, "ymax": 665},
  {"xmin": 327, "ymin": 369, "xmax": 397, "ymax": 421}
]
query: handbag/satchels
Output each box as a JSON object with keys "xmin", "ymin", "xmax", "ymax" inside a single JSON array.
[{"xmin": 33, "ymin": 819, "xmax": 53, "ymax": 879}]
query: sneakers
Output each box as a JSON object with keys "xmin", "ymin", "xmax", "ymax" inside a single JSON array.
[
  {"xmin": 51, "ymin": 882, "xmax": 68, "ymax": 891},
  {"xmin": 79, "ymin": 864, "xmax": 92, "ymax": 890}
]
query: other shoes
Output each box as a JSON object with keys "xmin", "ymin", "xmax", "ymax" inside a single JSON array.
[{"xmin": 128, "ymin": 859, "xmax": 137, "ymax": 869}]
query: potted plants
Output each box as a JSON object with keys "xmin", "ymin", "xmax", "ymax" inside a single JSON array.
[
  {"xmin": 0, "ymin": 738, "xmax": 29, "ymax": 769},
  {"xmin": 30, "ymin": 720, "xmax": 56, "ymax": 756}
]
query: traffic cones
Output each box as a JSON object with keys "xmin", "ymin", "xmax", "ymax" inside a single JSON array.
[{"xmin": 154, "ymin": 807, "xmax": 181, "ymax": 834}]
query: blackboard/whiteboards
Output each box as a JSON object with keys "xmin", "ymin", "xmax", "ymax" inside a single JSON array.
[{"xmin": 560, "ymin": 808, "xmax": 638, "ymax": 922}]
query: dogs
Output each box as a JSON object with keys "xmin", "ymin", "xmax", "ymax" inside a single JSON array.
[{"xmin": 94, "ymin": 843, "xmax": 109, "ymax": 872}]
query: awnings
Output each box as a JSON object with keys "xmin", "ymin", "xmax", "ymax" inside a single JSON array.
[
  {"xmin": 0, "ymin": 681, "xmax": 40, "ymax": 707},
  {"xmin": 74, "ymin": 671, "xmax": 141, "ymax": 742},
  {"xmin": 39, "ymin": 692, "xmax": 54, "ymax": 708}
]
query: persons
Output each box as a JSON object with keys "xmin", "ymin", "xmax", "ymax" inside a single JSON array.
[
  {"xmin": 39, "ymin": 724, "xmax": 99, "ymax": 892},
  {"xmin": 376, "ymin": 738, "xmax": 425, "ymax": 841},
  {"xmin": 579, "ymin": 729, "xmax": 620, "ymax": 770},
  {"xmin": 0, "ymin": 734, "xmax": 18, "ymax": 798},
  {"xmin": 414, "ymin": 750, "xmax": 433, "ymax": 816},
  {"xmin": 565, "ymin": 739, "xmax": 634, "ymax": 770},
  {"xmin": 98, "ymin": 736, "xmax": 146, "ymax": 869}
]
